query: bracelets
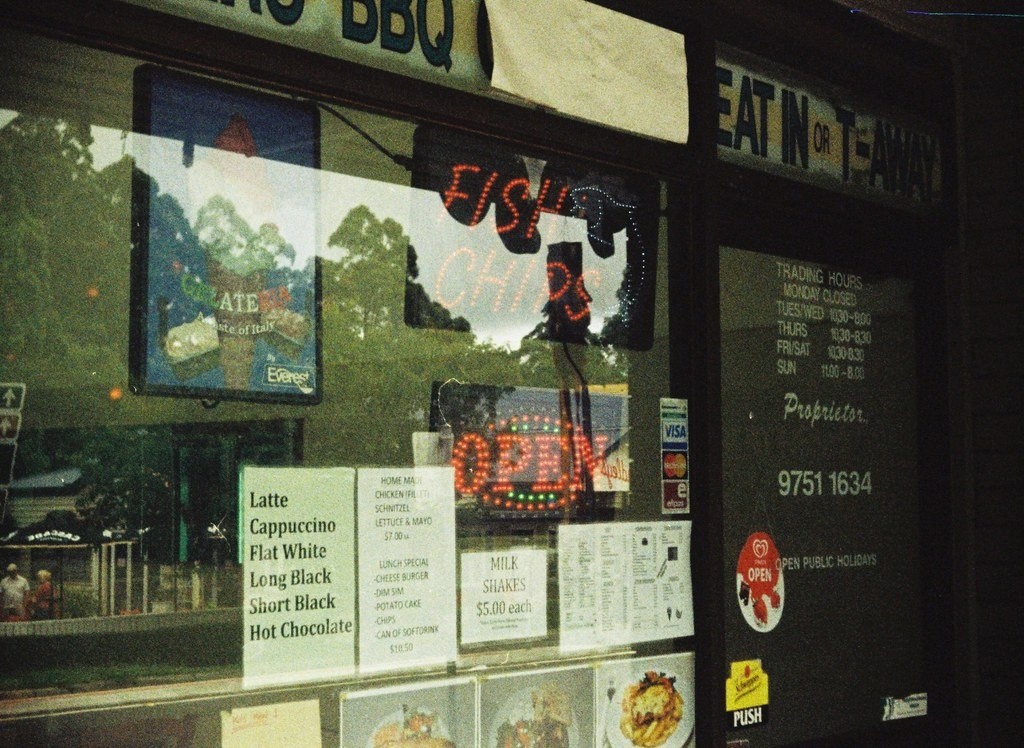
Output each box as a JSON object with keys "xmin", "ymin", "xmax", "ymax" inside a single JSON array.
[{"xmin": 24, "ymin": 604, "xmax": 28, "ymax": 608}]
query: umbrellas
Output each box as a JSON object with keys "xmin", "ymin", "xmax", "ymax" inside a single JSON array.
[{"xmin": 0, "ymin": 512, "xmax": 137, "ymax": 619}]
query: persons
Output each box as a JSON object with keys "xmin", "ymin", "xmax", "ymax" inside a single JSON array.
[
  {"xmin": 0, "ymin": 563, "xmax": 30, "ymax": 610},
  {"xmin": 32, "ymin": 569, "xmax": 57, "ymax": 620}
]
